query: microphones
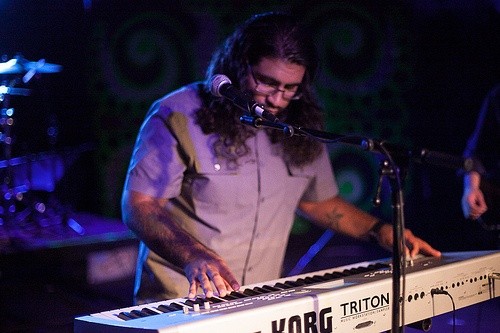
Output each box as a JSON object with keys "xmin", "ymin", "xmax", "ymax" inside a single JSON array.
[{"xmin": 207, "ymin": 73, "xmax": 279, "ymax": 124}]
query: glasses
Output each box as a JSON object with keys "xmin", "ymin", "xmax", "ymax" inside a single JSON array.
[{"xmin": 248, "ymin": 63, "xmax": 303, "ymax": 99}]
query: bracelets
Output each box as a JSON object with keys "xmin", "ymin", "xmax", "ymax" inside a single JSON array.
[{"xmin": 369, "ymin": 220, "xmax": 389, "ymax": 249}]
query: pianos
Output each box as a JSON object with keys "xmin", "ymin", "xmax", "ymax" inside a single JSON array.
[{"xmin": 74, "ymin": 249, "xmax": 500, "ymax": 333}]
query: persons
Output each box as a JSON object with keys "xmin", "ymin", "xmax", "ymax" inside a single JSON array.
[
  {"xmin": 121, "ymin": 11, "xmax": 442, "ymax": 306},
  {"xmin": 458, "ymin": 84, "xmax": 500, "ymax": 250}
]
